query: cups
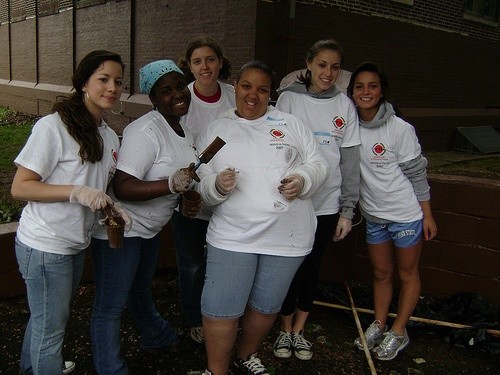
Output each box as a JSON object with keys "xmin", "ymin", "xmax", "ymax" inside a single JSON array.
[
  {"xmin": 105, "ymin": 217, "xmax": 125, "ymax": 248},
  {"xmin": 179, "ymin": 191, "xmax": 201, "ymax": 217}
]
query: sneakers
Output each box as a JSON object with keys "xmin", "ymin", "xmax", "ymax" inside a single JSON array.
[
  {"xmin": 290, "ymin": 330, "xmax": 312, "ymax": 360},
  {"xmin": 233, "ymin": 350, "xmax": 268, "ymax": 375},
  {"xmin": 271, "ymin": 328, "xmax": 291, "ymax": 358},
  {"xmin": 353, "ymin": 320, "xmax": 389, "ymax": 350},
  {"xmin": 373, "ymin": 327, "xmax": 409, "ymax": 361}
]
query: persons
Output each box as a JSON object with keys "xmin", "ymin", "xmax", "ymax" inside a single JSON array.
[
  {"xmin": 91, "ymin": 59, "xmax": 200, "ymax": 375},
  {"xmin": 272, "ymin": 40, "xmax": 360, "ymax": 360},
  {"xmin": 11, "ymin": 49, "xmax": 126, "ymax": 375},
  {"xmin": 195, "ymin": 61, "xmax": 329, "ymax": 375},
  {"xmin": 180, "ymin": 37, "xmax": 240, "ymax": 145},
  {"xmin": 346, "ymin": 60, "xmax": 438, "ymax": 360}
]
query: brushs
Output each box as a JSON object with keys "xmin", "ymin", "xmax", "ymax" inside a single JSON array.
[
  {"xmin": 191, "ymin": 136, "xmax": 226, "ymax": 173},
  {"xmin": 106, "ymin": 206, "xmax": 119, "ymax": 226}
]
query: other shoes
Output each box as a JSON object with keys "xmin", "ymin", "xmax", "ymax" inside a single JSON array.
[
  {"xmin": 144, "ymin": 337, "xmax": 194, "ymax": 354},
  {"xmin": 190, "ymin": 326, "xmax": 206, "ymax": 343},
  {"xmin": 62, "ymin": 360, "xmax": 76, "ymax": 374}
]
